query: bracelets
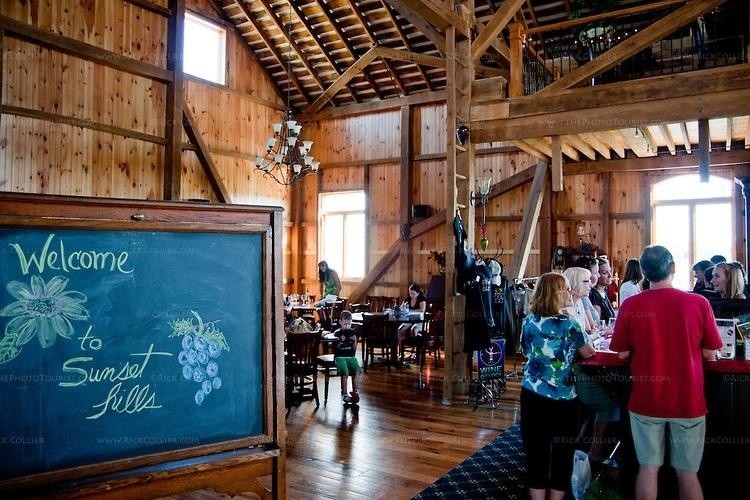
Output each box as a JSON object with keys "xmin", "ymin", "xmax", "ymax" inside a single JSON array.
[{"xmin": 320, "ymin": 295, "xmax": 323, "ymax": 297}]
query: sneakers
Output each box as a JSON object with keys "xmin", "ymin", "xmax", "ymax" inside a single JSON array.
[
  {"xmin": 352, "ymin": 392, "xmax": 360, "ymax": 403},
  {"xmin": 343, "ymin": 396, "xmax": 351, "ymax": 402}
]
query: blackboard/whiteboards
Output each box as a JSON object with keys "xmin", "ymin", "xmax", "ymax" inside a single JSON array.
[{"xmin": 0, "ymin": 227, "xmax": 271, "ymax": 480}]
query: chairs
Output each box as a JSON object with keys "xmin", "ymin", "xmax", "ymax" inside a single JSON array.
[
  {"xmin": 583, "ymin": 34, "xmax": 621, "ymax": 86},
  {"xmin": 285, "ymin": 294, "xmax": 445, "ymax": 419},
  {"xmin": 691, "ymin": 16, "xmax": 745, "ymax": 70}
]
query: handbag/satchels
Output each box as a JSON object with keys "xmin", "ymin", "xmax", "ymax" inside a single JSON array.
[
  {"xmin": 583, "ymin": 479, "xmax": 624, "ymax": 500},
  {"xmin": 571, "ymin": 450, "xmax": 591, "ymax": 500}
]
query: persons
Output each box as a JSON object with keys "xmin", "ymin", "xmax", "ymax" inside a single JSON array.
[
  {"xmin": 515, "ymin": 272, "xmax": 596, "ymax": 500},
  {"xmin": 318, "ymin": 260, "xmax": 342, "ymax": 301},
  {"xmin": 332, "ymin": 310, "xmax": 361, "ymax": 403},
  {"xmin": 608, "ymin": 244, "xmax": 724, "ymax": 500},
  {"xmin": 396, "ymin": 283, "xmax": 426, "ymax": 361},
  {"xmin": 563, "ymin": 256, "xmax": 750, "ymax": 469}
]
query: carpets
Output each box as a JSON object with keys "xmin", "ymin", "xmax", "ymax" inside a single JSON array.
[{"xmin": 411, "ymin": 419, "xmax": 532, "ymax": 499}]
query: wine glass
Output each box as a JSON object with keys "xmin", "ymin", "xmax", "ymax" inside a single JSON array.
[{"xmin": 283, "ymin": 293, "xmax": 313, "ymax": 309}]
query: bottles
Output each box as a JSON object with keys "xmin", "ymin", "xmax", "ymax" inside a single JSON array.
[
  {"xmin": 456, "ymin": 121, "xmax": 470, "ymax": 146},
  {"xmin": 384, "ymin": 299, "xmax": 410, "ymax": 319}
]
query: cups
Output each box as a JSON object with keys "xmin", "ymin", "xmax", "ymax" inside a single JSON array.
[{"xmin": 587, "ymin": 317, "xmax": 618, "ymax": 353}]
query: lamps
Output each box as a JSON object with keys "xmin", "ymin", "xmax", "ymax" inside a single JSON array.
[
  {"xmin": 255, "ymin": 4, "xmax": 321, "ymax": 185},
  {"xmin": 575, "ymin": 222, "xmax": 587, "ymax": 236},
  {"xmin": 470, "ymin": 176, "xmax": 492, "ymax": 205}
]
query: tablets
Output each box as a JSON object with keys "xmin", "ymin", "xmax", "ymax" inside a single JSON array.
[{"xmin": 344, "ymin": 329, "xmax": 355, "ymax": 342}]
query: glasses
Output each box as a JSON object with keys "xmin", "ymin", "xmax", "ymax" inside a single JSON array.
[
  {"xmin": 558, "ymin": 286, "xmax": 571, "ymax": 292},
  {"xmin": 583, "ymin": 279, "xmax": 590, "ymax": 283}
]
